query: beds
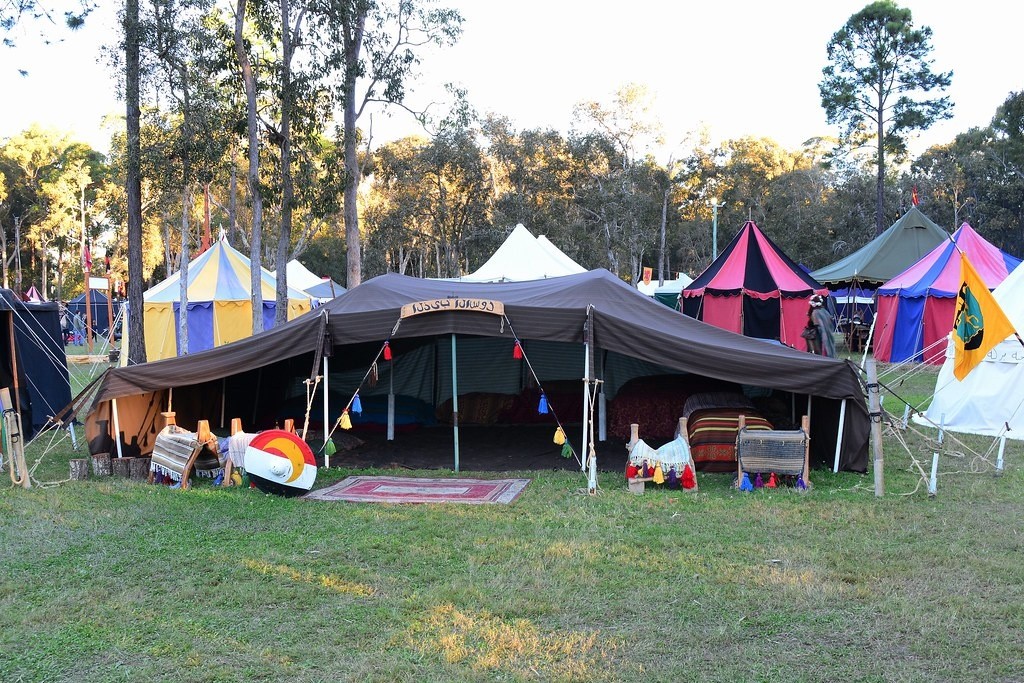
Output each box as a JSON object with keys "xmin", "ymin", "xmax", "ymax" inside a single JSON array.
[
  {"xmin": 606, "ymin": 373, "xmax": 743, "ymax": 440},
  {"xmin": 682, "ymin": 393, "xmax": 772, "ymax": 474}
]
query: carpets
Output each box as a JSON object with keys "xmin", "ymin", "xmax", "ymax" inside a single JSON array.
[{"xmin": 297, "ymin": 474, "xmax": 536, "ymax": 506}]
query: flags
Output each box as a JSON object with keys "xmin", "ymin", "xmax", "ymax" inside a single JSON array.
[
  {"xmin": 642, "ymin": 267, "xmax": 653, "ymax": 286},
  {"xmin": 951, "ymin": 253, "xmax": 1015, "ymax": 383},
  {"xmin": 86, "ymin": 249, "xmax": 92, "ymax": 271},
  {"xmin": 912, "ymin": 183, "xmax": 920, "ymax": 206},
  {"xmin": 106, "ymin": 252, "xmax": 111, "ymax": 274}
]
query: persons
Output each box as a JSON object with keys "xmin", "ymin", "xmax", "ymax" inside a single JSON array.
[
  {"xmin": 800, "ymin": 294, "xmax": 838, "ymax": 359},
  {"xmin": 73, "ymin": 310, "xmax": 86, "ymax": 346},
  {"xmin": 60, "ymin": 310, "xmax": 70, "ymax": 346},
  {"xmin": 92, "ymin": 320, "xmax": 98, "ymax": 343}
]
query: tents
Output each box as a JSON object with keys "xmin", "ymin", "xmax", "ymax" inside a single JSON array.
[{"xmin": 0, "ymin": 205, "xmax": 1024, "ymax": 502}]
